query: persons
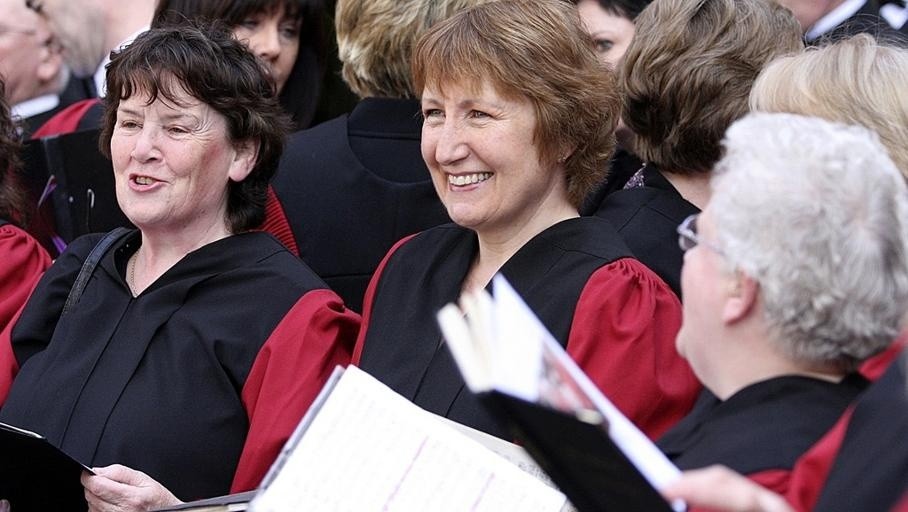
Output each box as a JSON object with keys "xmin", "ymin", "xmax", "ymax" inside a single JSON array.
[{"xmin": 2, "ymin": 0, "xmax": 908, "ymax": 512}]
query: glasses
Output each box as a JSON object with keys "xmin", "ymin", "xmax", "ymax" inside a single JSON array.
[{"xmin": 676, "ymin": 215, "xmax": 730, "ymax": 257}]
